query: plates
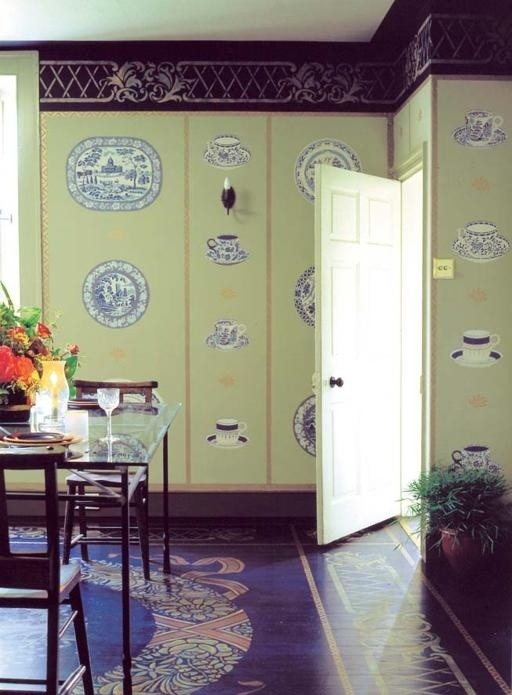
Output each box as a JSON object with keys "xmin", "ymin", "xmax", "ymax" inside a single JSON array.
[
  {"xmin": 0, "ymin": 434, "xmax": 84, "ymax": 446},
  {"xmin": 293, "ymin": 139, "xmax": 361, "ymax": 457},
  {"xmin": 65, "ymin": 136, "xmax": 162, "ymax": 473},
  {"xmin": 2, "ymin": 433, "xmax": 71, "ymax": 442}
]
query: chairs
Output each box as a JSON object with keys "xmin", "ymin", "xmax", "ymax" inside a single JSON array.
[
  {"xmin": 62, "ymin": 379, "xmax": 158, "ymax": 578},
  {"xmin": 0, "ymin": 445, "xmax": 95, "ymax": 694}
]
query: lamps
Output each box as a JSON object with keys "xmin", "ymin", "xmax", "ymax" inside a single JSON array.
[{"xmin": 220, "ymin": 177, "xmax": 236, "ymax": 215}]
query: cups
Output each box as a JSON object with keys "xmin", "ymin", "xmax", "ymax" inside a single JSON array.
[
  {"xmin": 206, "ymin": 135, "xmax": 246, "ymax": 445},
  {"xmin": 30, "ymin": 361, "xmax": 69, "ymax": 435},
  {"xmin": 450, "ymin": 109, "xmax": 503, "ymax": 471}
]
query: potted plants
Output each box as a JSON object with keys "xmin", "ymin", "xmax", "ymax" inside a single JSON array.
[{"xmin": 387, "ymin": 457, "xmax": 512, "ymax": 571}]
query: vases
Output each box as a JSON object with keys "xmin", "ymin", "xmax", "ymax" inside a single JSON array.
[{"xmin": 1, "ymin": 386, "xmax": 31, "ymax": 422}]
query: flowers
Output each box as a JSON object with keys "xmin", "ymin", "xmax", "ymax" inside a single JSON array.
[{"xmin": 1, "ymin": 279, "xmax": 80, "ymax": 389}]
